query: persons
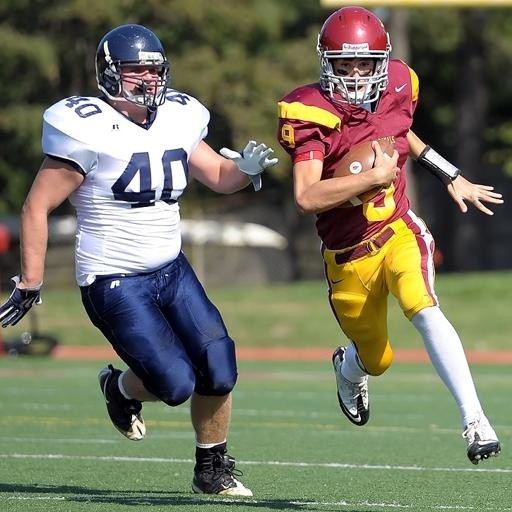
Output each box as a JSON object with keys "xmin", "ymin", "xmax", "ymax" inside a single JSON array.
[
  {"xmin": 276, "ymin": 5, "xmax": 506, "ymax": 466},
  {"xmin": 0, "ymin": 23, "xmax": 279, "ymax": 498}
]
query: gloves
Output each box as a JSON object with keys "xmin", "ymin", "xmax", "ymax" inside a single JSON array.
[
  {"xmin": 0, "ymin": 272, "xmax": 44, "ymax": 328},
  {"xmin": 219, "ymin": 138, "xmax": 279, "ymax": 193}
]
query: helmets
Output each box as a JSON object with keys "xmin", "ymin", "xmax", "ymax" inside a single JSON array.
[
  {"xmin": 315, "ymin": 5, "xmax": 392, "ymax": 102},
  {"xmin": 94, "ymin": 23, "xmax": 169, "ymax": 103}
]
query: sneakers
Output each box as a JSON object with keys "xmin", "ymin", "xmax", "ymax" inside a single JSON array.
[
  {"xmin": 190, "ymin": 442, "xmax": 254, "ymax": 498},
  {"xmin": 97, "ymin": 363, "xmax": 147, "ymax": 441},
  {"xmin": 331, "ymin": 343, "xmax": 370, "ymax": 426},
  {"xmin": 462, "ymin": 415, "xmax": 502, "ymax": 466}
]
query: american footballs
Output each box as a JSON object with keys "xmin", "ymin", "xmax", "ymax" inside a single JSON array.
[{"xmin": 334, "ymin": 136, "xmax": 396, "ymax": 209}]
